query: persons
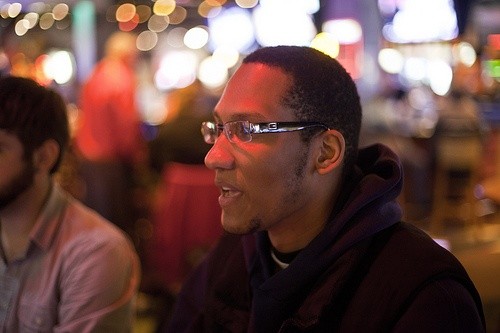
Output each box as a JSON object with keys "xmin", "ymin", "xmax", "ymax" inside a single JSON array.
[
  {"xmin": 166, "ymin": 44, "xmax": 486, "ymax": 333},
  {"xmin": 75, "ymin": 32, "xmax": 146, "ymax": 231},
  {"xmin": 0, "ymin": 74, "xmax": 141, "ymax": 333}
]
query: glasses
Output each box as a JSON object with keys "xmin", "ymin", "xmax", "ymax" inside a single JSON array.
[{"xmin": 199, "ymin": 119, "xmax": 331, "ymax": 146}]
